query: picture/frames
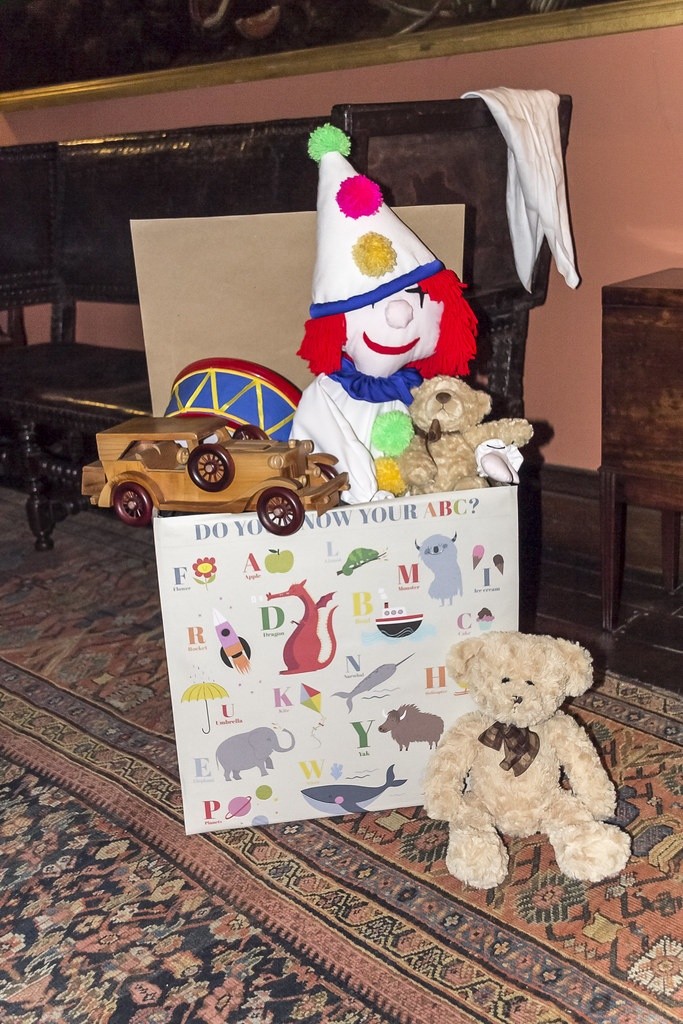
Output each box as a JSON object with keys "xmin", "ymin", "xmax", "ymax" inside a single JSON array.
[{"xmin": 0, "ymin": 0, "xmax": 683, "ymax": 113}]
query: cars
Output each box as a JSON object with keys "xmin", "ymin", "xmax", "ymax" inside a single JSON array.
[{"xmin": 82, "ymin": 416, "xmax": 351, "ymax": 536}]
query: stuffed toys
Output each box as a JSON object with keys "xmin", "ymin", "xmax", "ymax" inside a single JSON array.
[
  {"xmin": 424, "ymin": 630, "xmax": 630, "ymax": 888},
  {"xmin": 291, "ymin": 123, "xmax": 524, "ymax": 504},
  {"xmin": 399, "ymin": 375, "xmax": 535, "ymax": 494}
]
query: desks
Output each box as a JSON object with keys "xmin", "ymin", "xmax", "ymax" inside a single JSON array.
[{"xmin": 597, "ymin": 267, "xmax": 683, "ymax": 631}]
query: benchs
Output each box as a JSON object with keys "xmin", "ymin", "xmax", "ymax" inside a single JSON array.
[{"xmin": 0, "ymin": 92, "xmax": 574, "ymax": 553}]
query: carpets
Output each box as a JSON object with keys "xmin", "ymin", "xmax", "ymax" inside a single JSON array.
[{"xmin": 0, "ymin": 485, "xmax": 683, "ymax": 1024}]
